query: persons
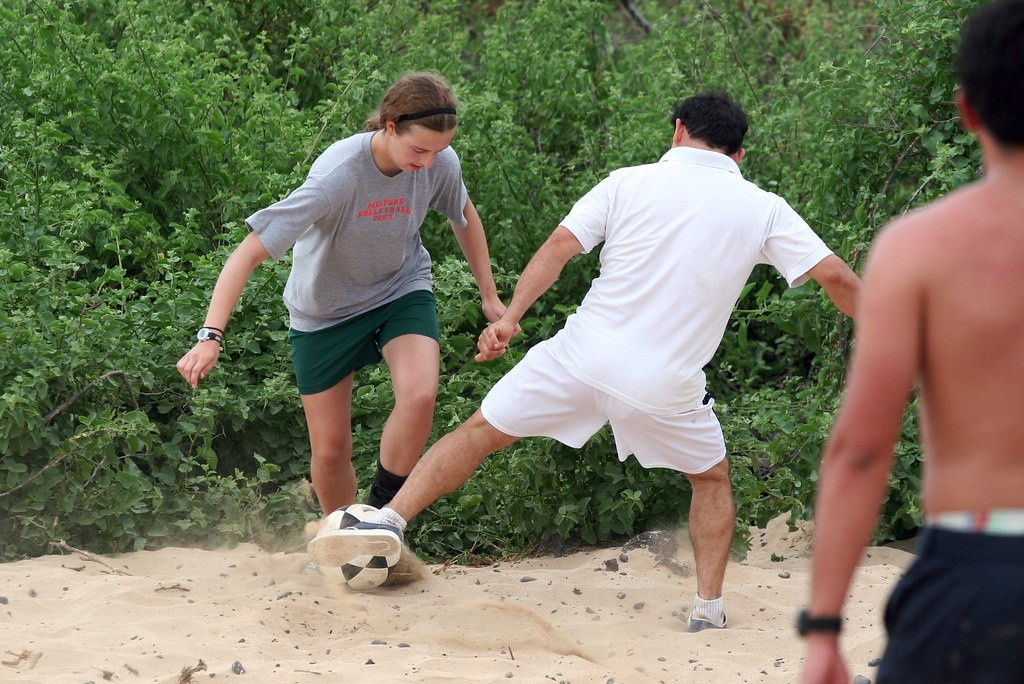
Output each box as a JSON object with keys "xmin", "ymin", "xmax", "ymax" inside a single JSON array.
[
  {"xmin": 307, "ymin": 91, "xmax": 863, "ymax": 634},
  {"xmin": 795, "ymin": 1, "xmax": 1023, "ymax": 684},
  {"xmin": 175, "ymin": 73, "xmax": 522, "ymax": 581}
]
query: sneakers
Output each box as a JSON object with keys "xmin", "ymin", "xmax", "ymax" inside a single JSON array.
[
  {"xmin": 687, "ymin": 611, "xmax": 727, "ymax": 632},
  {"xmin": 306, "ymin": 513, "xmax": 401, "ymax": 568}
]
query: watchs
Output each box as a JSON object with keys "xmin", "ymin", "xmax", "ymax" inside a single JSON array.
[
  {"xmin": 798, "ymin": 612, "xmax": 841, "ymax": 636},
  {"xmin": 196, "ymin": 328, "xmax": 222, "ymax": 343}
]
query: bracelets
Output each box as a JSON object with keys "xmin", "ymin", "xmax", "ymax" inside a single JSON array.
[{"xmin": 202, "ymin": 327, "xmax": 223, "ymax": 335}]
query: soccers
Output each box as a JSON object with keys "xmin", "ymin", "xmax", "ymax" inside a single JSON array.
[{"xmin": 314, "ymin": 503, "xmax": 402, "ymax": 591}]
